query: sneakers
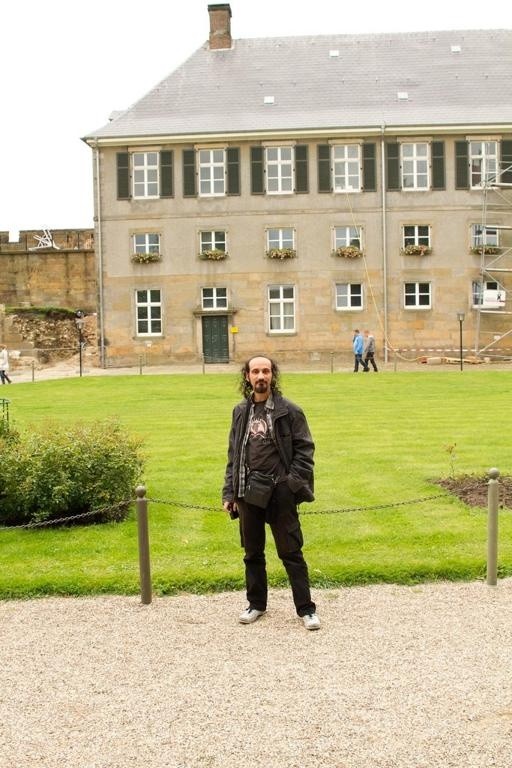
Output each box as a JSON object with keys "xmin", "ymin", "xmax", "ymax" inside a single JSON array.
[
  {"xmin": 303, "ymin": 613, "xmax": 321, "ymax": 630},
  {"xmin": 238, "ymin": 608, "xmax": 267, "ymax": 624}
]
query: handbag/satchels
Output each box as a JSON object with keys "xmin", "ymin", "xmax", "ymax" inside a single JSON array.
[{"xmin": 241, "ymin": 470, "xmax": 276, "ymax": 508}]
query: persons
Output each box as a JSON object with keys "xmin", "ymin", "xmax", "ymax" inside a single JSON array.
[
  {"xmin": 1, "ymin": 342, "xmax": 15, "ymax": 386},
  {"xmin": 219, "ymin": 351, "xmax": 335, "ymax": 631},
  {"xmin": 360, "ymin": 328, "xmax": 378, "ymax": 373},
  {"xmin": 351, "ymin": 328, "xmax": 370, "ymax": 372}
]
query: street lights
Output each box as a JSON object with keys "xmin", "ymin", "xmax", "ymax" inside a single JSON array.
[
  {"xmin": 456, "ymin": 313, "xmax": 465, "ymax": 372},
  {"xmin": 75, "ymin": 310, "xmax": 84, "ymax": 377}
]
